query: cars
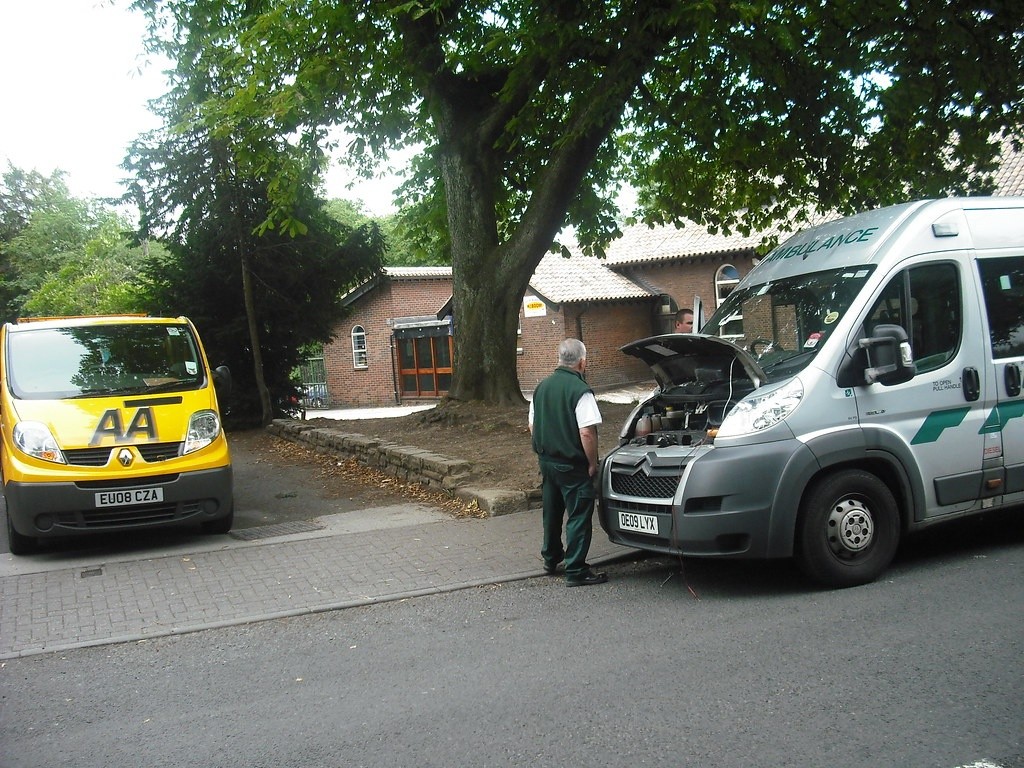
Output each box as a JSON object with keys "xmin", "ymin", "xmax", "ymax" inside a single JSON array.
[{"xmin": 300, "ymin": 383, "xmax": 328, "ymax": 409}]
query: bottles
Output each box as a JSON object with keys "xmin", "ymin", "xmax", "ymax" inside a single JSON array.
[{"xmin": 634, "ymin": 413, "xmax": 651, "ymax": 437}]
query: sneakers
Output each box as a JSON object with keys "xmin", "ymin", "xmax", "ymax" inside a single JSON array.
[
  {"xmin": 542, "ymin": 556, "xmax": 590, "ymax": 571},
  {"xmin": 566, "ymin": 566, "xmax": 608, "ymax": 587}
]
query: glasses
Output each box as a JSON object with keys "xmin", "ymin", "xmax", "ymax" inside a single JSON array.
[{"xmin": 680, "ymin": 322, "xmax": 693, "ymax": 325}]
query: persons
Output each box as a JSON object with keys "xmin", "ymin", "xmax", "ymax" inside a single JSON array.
[
  {"xmin": 673, "ymin": 309, "xmax": 694, "ymax": 334},
  {"xmin": 528, "ymin": 338, "xmax": 608, "ymax": 588}
]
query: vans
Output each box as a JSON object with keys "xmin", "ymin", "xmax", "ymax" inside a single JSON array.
[
  {"xmin": 0, "ymin": 312, "xmax": 236, "ymax": 557},
  {"xmin": 596, "ymin": 195, "xmax": 1023, "ymax": 589}
]
